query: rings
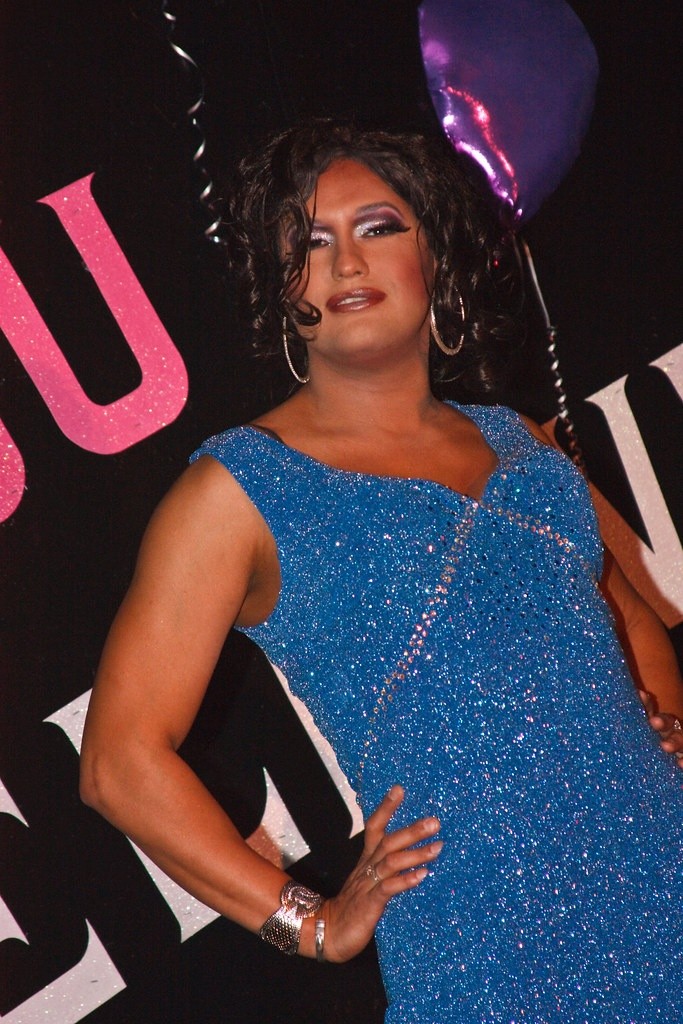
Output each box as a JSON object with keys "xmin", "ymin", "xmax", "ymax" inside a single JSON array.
[
  {"xmin": 674, "ymin": 718, "xmax": 682, "ymax": 730},
  {"xmin": 365, "ymin": 865, "xmax": 400, "ymax": 882}
]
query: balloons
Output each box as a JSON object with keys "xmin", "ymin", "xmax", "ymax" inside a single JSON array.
[{"xmin": 418, "ymin": 1, "xmax": 597, "ymax": 228}]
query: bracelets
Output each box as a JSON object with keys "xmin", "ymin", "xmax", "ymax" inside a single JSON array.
[{"xmin": 257, "ymin": 880, "xmax": 324, "ymax": 962}]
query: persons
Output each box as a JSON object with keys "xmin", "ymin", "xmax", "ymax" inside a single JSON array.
[{"xmin": 81, "ymin": 100, "xmax": 683, "ymax": 1024}]
